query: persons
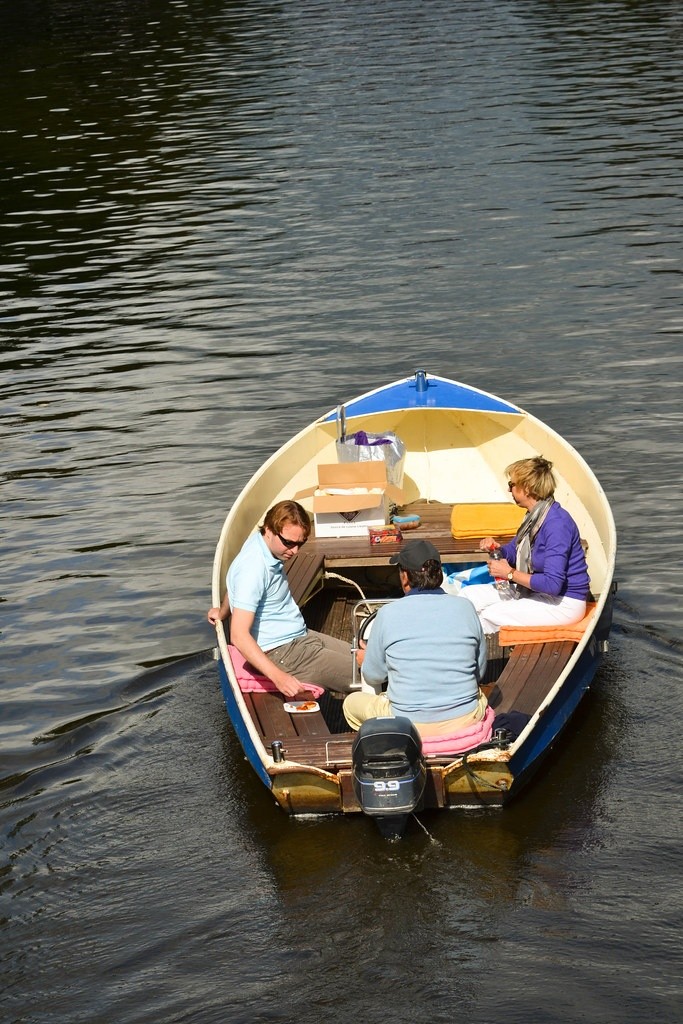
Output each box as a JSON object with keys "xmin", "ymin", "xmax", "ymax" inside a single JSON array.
[
  {"xmin": 457, "ymin": 454, "xmax": 591, "ymax": 639},
  {"xmin": 342, "ymin": 540, "xmax": 488, "ymax": 737},
  {"xmin": 208, "ymin": 500, "xmax": 362, "ymax": 697}
]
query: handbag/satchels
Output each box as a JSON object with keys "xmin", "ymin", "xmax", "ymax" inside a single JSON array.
[{"xmin": 336, "ymin": 431, "xmax": 407, "ymax": 490}]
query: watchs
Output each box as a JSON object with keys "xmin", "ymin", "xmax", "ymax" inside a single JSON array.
[{"xmin": 507, "ymin": 569, "xmax": 515, "ymax": 581}]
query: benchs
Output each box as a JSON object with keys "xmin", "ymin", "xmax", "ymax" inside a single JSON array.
[{"xmin": 227, "ymin": 504, "xmax": 589, "ymax": 770}]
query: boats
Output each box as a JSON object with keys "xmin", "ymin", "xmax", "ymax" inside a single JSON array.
[{"xmin": 212, "ymin": 368, "xmax": 616, "ymax": 818}]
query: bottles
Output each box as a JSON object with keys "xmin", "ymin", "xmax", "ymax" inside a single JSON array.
[{"xmin": 490, "ymin": 544, "xmax": 510, "ymax": 589}]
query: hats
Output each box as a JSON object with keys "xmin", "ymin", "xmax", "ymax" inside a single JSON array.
[{"xmin": 389, "ymin": 540, "xmax": 441, "ymax": 571}]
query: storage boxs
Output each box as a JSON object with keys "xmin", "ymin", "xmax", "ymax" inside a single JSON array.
[{"xmin": 293, "ymin": 461, "xmax": 421, "ymax": 538}]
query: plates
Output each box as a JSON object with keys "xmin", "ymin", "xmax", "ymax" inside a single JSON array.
[{"xmin": 283, "ymin": 701, "xmax": 320, "ymax": 713}]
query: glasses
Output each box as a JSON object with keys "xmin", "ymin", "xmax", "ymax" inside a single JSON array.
[
  {"xmin": 508, "ymin": 481, "xmax": 516, "ymax": 488},
  {"xmin": 276, "ymin": 531, "xmax": 306, "ymax": 550}
]
